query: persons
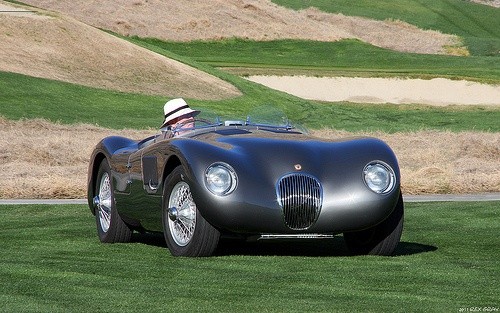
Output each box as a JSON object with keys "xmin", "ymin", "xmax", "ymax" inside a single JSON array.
[{"xmin": 159, "ymin": 98, "xmax": 201, "ymax": 138}]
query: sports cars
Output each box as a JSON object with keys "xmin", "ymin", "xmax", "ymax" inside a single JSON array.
[{"xmin": 88, "ymin": 110, "xmax": 405, "ymax": 258}]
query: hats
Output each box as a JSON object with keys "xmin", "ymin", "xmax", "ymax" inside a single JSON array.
[{"xmin": 159, "ymin": 97, "xmax": 201, "ymax": 129}]
